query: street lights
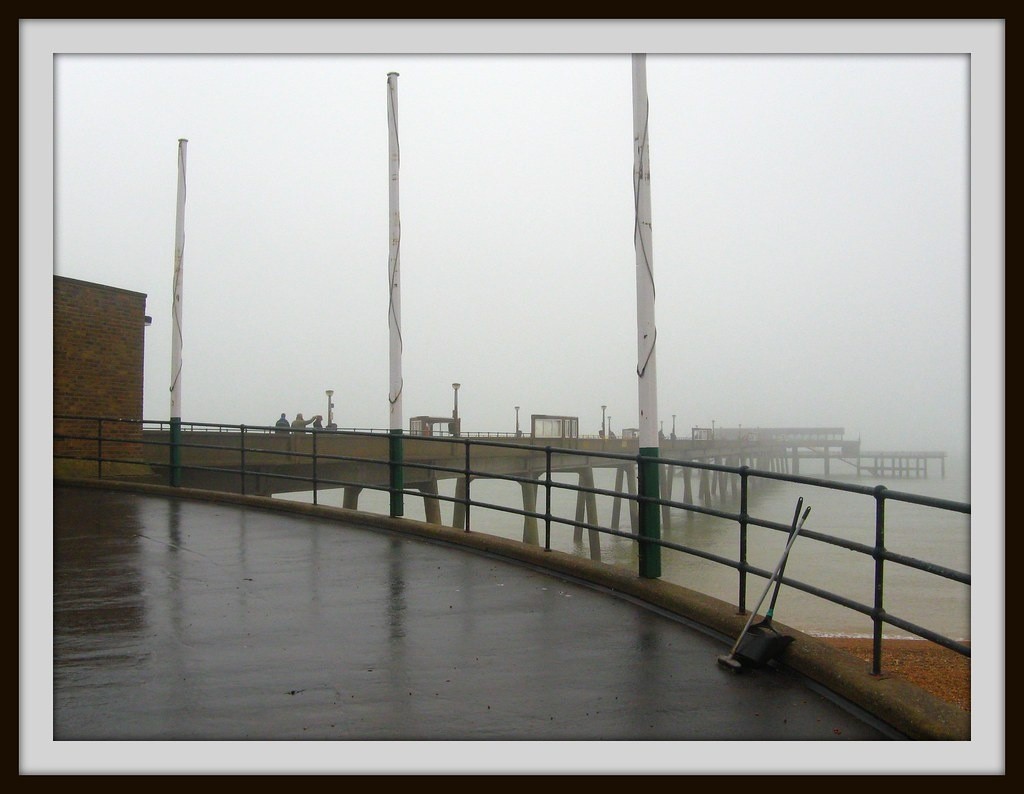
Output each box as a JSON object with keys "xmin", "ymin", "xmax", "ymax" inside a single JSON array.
[
  {"xmin": 326, "ymin": 390, "xmax": 333, "ymax": 426},
  {"xmin": 515, "ymin": 406, "xmax": 520, "ymax": 433},
  {"xmin": 672, "ymin": 415, "xmax": 676, "ymax": 433},
  {"xmin": 452, "ymin": 383, "xmax": 460, "ymax": 437},
  {"xmin": 601, "ymin": 406, "xmax": 607, "ymax": 436},
  {"xmin": 608, "ymin": 416, "xmax": 611, "ymax": 435}
]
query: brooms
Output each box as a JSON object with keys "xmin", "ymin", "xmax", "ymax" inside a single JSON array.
[{"xmin": 717, "ymin": 497, "xmax": 812, "ymax": 675}]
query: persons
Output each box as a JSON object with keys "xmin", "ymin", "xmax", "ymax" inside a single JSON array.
[
  {"xmin": 291, "ymin": 414, "xmax": 317, "ymax": 433},
  {"xmin": 275, "ymin": 413, "xmax": 290, "ymax": 434},
  {"xmin": 658, "ymin": 431, "xmax": 666, "ymax": 440},
  {"xmin": 313, "ymin": 415, "xmax": 322, "ymax": 428}
]
